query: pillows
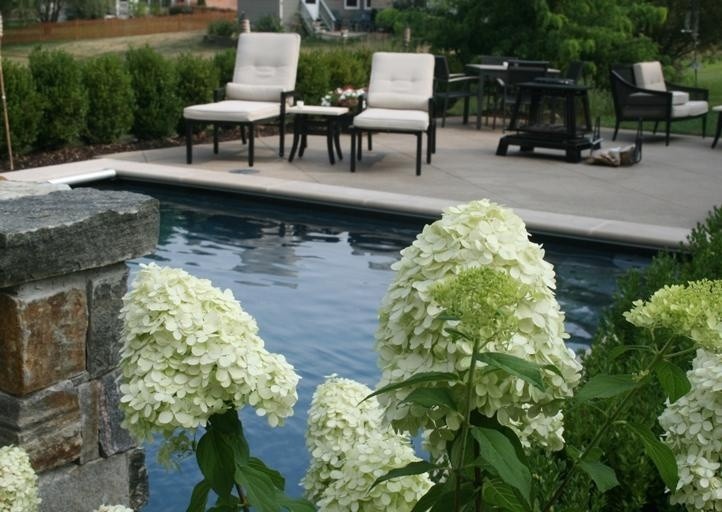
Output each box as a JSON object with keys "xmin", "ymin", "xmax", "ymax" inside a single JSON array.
[
  {"xmin": 628, "ymin": 90, "xmax": 688, "ymax": 106},
  {"xmin": 632, "ymin": 61, "xmax": 667, "ymax": 92}
]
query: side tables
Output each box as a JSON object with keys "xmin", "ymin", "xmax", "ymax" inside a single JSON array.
[
  {"xmin": 285, "ymin": 104, "xmax": 349, "ymax": 165},
  {"xmin": 710, "ymin": 105, "xmax": 722, "ymax": 149}
]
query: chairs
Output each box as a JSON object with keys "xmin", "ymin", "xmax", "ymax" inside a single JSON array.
[
  {"xmin": 434, "ymin": 54, "xmax": 589, "ymax": 136},
  {"xmin": 350, "ymin": 51, "xmax": 436, "ymax": 176},
  {"xmin": 181, "ymin": 31, "xmax": 302, "ymax": 167},
  {"xmin": 609, "ymin": 64, "xmax": 710, "ymax": 146}
]
